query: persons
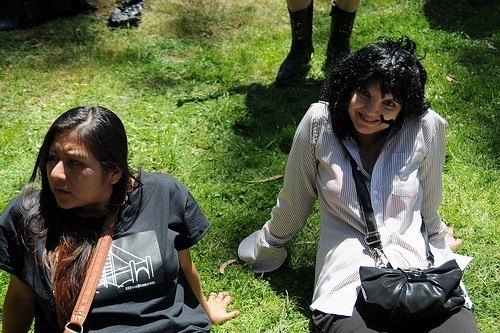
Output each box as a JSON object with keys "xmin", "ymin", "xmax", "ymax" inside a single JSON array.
[
  {"xmin": 276, "ymin": 0, "xmax": 360, "ymax": 83},
  {"xmin": 236, "ymin": 29, "xmax": 480, "ymax": 332},
  {"xmin": 1, "ymin": 104, "xmax": 239, "ymax": 333}
]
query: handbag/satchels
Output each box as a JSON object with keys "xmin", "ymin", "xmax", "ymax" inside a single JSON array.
[{"xmin": 359, "ymin": 259, "xmax": 466, "ymax": 333}]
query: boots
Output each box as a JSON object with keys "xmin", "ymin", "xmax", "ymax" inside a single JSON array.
[
  {"xmin": 276, "ymin": 0, "xmax": 314, "ymax": 82},
  {"xmin": 326, "ymin": 0, "xmax": 357, "ymax": 63}
]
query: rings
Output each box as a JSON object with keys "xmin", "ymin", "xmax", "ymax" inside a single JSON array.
[
  {"xmin": 208, "ymin": 294, "xmax": 217, "ymax": 298},
  {"xmin": 216, "ymin": 295, "xmax": 223, "ymax": 300}
]
